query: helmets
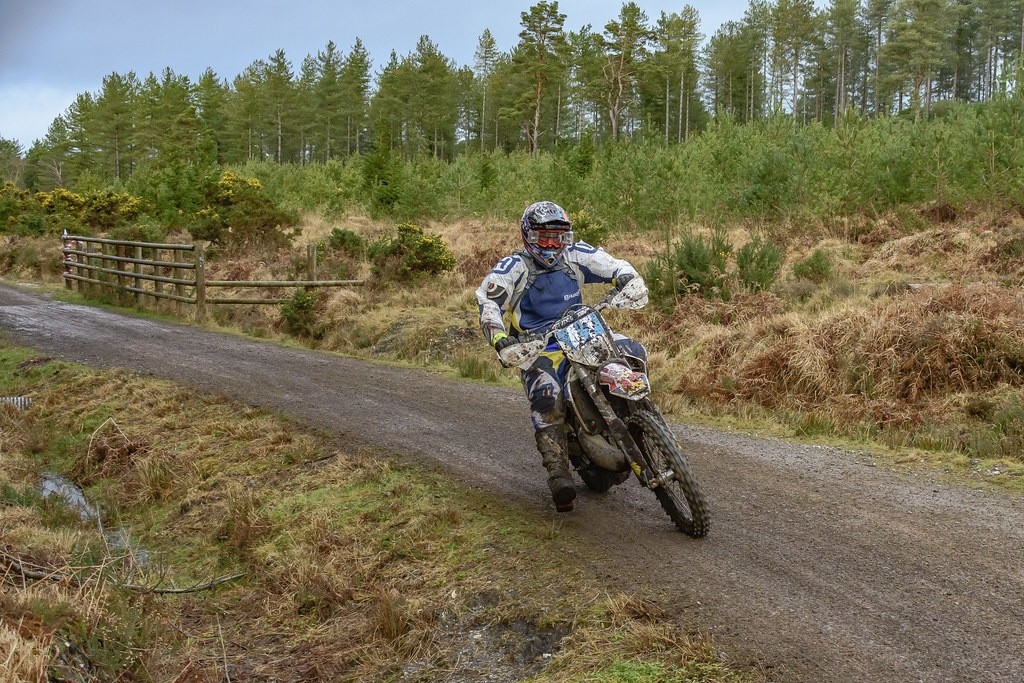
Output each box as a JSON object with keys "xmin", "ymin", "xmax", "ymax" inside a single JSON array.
[{"xmin": 520, "ymin": 201, "xmax": 574, "ymax": 267}]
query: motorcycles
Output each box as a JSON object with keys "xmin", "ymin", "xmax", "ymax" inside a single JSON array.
[{"xmin": 497, "ymin": 275, "xmax": 712, "ymax": 540}]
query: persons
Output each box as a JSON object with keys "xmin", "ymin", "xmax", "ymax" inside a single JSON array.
[{"xmin": 476, "ymin": 201, "xmax": 650, "ymax": 511}]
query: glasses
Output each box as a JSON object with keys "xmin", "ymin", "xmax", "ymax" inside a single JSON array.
[{"xmin": 532, "ymin": 229, "xmax": 567, "ymax": 247}]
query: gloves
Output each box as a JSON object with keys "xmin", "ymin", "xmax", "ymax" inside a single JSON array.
[
  {"xmin": 495, "ymin": 335, "xmax": 518, "ymax": 354},
  {"xmin": 615, "ymin": 273, "xmax": 634, "ymax": 292}
]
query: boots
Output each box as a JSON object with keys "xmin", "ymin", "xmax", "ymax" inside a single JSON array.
[{"xmin": 535, "ymin": 424, "xmax": 576, "ymax": 511}]
